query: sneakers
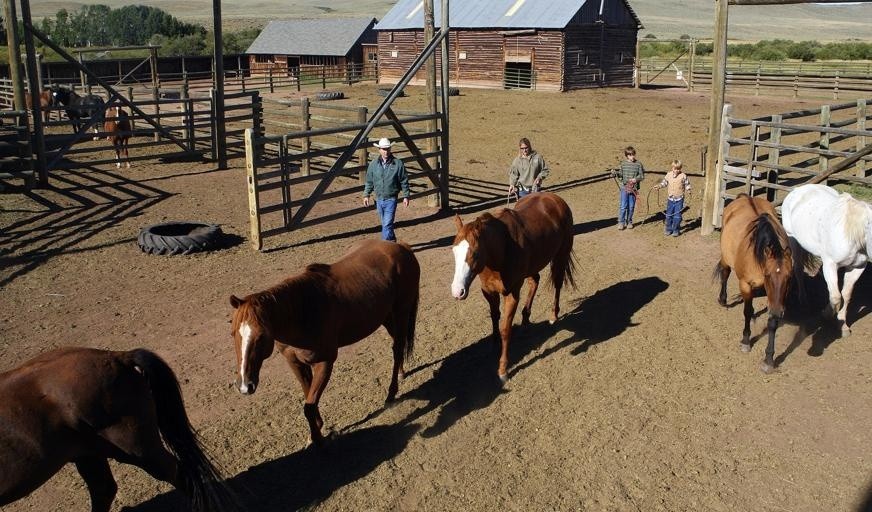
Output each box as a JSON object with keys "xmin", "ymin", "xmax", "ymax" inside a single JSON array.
[
  {"xmin": 618, "ymin": 223, "xmax": 624, "ymax": 230},
  {"xmin": 672, "ymin": 232, "xmax": 680, "ymax": 237},
  {"xmin": 664, "ymin": 231, "xmax": 672, "ymax": 236},
  {"xmin": 627, "ymin": 222, "xmax": 633, "ymax": 229}
]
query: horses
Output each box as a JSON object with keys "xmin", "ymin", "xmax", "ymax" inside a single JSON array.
[
  {"xmin": 780, "ymin": 184, "xmax": 867, "ymax": 337},
  {"xmin": 55, "ymin": 84, "xmax": 105, "ymax": 141},
  {"xmin": 0, "ymin": 348, "xmax": 228, "ymax": 512},
  {"xmin": 451, "ymin": 193, "xmax": 584, "ymax": 383},
  {"xmin": 229, "ymin": 239, "xmax": 431, "ymax": 458},
  {"xmin": 712, "ymin": 193, "xmax": 792, "ymax": 373},
  {"xmin": 106, "ymin": 104, "xmax": 135, "ymax": 169},
  {"xmin": 12, "ymin": 86, "xmax": 56, "ymax": 131}
]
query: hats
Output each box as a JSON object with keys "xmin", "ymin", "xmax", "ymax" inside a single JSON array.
[{"xmin": 372, "ymin": 138, "xmax": 396, "ymax": 149}]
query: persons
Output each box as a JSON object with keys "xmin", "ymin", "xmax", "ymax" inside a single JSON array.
[
  {"xmin": 508, "ymin": 137, "xmax": 549, "ymax": 203},
  {"xmin": 651, "ymin": 160, "xmax": 694, "ymax": 237},
  {"xmin": 363, "ymin": 138, "xmax": 411, "ymax": 241},
  {"xmin": 609, "ymin": 147, "xmax": 645, "ymax": 230}
]
